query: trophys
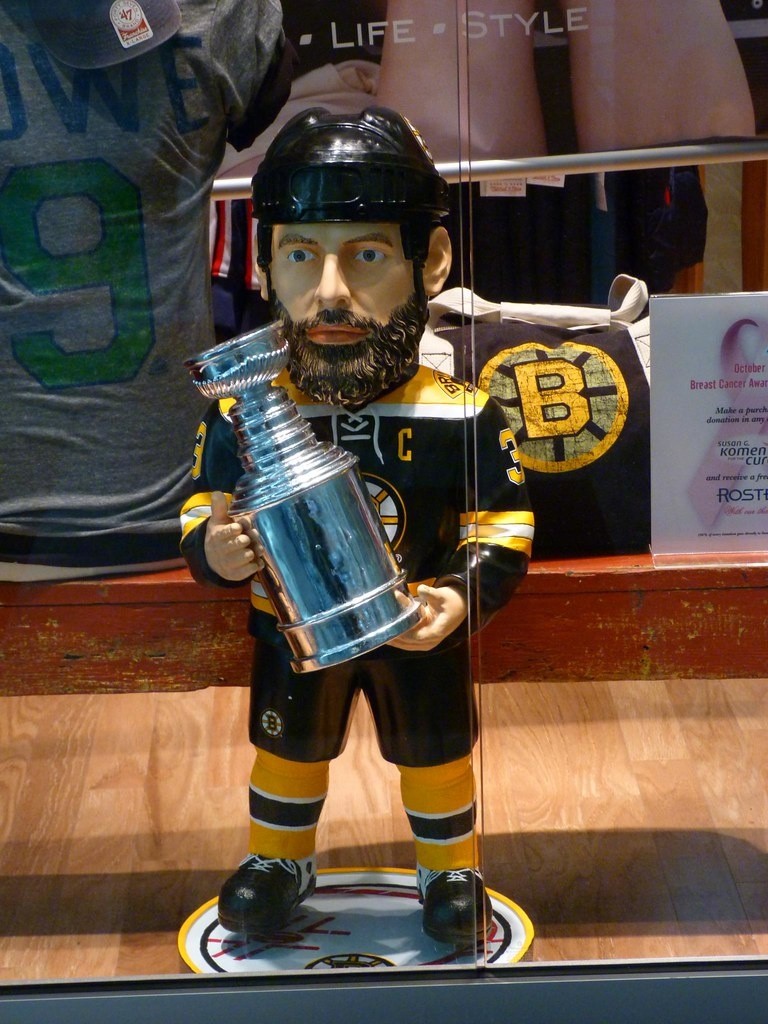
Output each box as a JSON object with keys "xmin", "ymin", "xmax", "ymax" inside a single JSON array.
[{"xmin": 185, "ymin": 316, "xmax": 425, "ymax": 676}]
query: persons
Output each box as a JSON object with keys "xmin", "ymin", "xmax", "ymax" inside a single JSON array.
[
  {"xmin": 178, "ymin": 107, "xmax": 535, "ymax": 941},
  {"xmin": 0, "ymin": 0, "xmax": 295, "ymax": 538},
  {"xmin": 378, "ymin": 0, "xmax": 756, "ymax": 163}
]
